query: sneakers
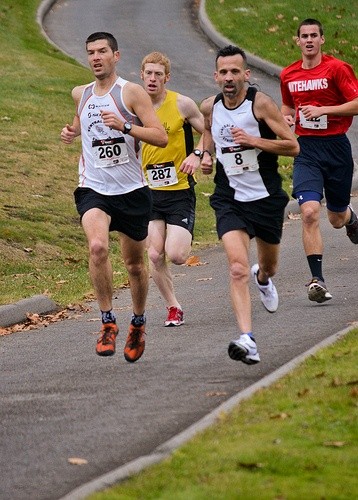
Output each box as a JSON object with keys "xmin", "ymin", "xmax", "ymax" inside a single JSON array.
[
  {"xmin": 345, "ymin": 204, "xmax": 358, "ymax": 244},
  {"xmin": 304, "ymin": 276, "xmax": 332, "ymax": 303},
  {"xmin": 227, "ymin": 334, "xmax": 260, "ymax": 365},
  {"xmin": 124, "ymin": 318, "xmax": 146, "ymax": 362},
  {"xmin": 251, "ymin": 264, "xmax": 279, "ymax": 313},
  {"xmin": 96, "ymin": 323, "xmax": 119, "ymax": 356},
  {"xmin": 164, "ymin": 306, "xmax": 184, "ymax": 326}
]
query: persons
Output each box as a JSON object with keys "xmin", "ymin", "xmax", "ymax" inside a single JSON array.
[
  {"xmin": 136, "ymin": 52, "xmax": 205, "ymax": 328},
  {"xmin": 59, "ymin": 32, "xmax": 168, "ymax": 364},
  {"xmin": 278, "ymin": 19, "xmax": 358, "ymax": 303},
  {"xmin": 199, "ymin": 45, "xmax": 301, "ymax": 366}
]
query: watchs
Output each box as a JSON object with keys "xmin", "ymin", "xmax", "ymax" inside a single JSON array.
[
  {"xmin": 122, "ymin": 122, "xmax": 132, "ymax": 134},
  {"xmin": 192, "ymin": 149, "xmax": 201, "ymax": 156},
  {"xmin": 199, "ymin": 150, "xmax": 211, "ymax": 159}
]
